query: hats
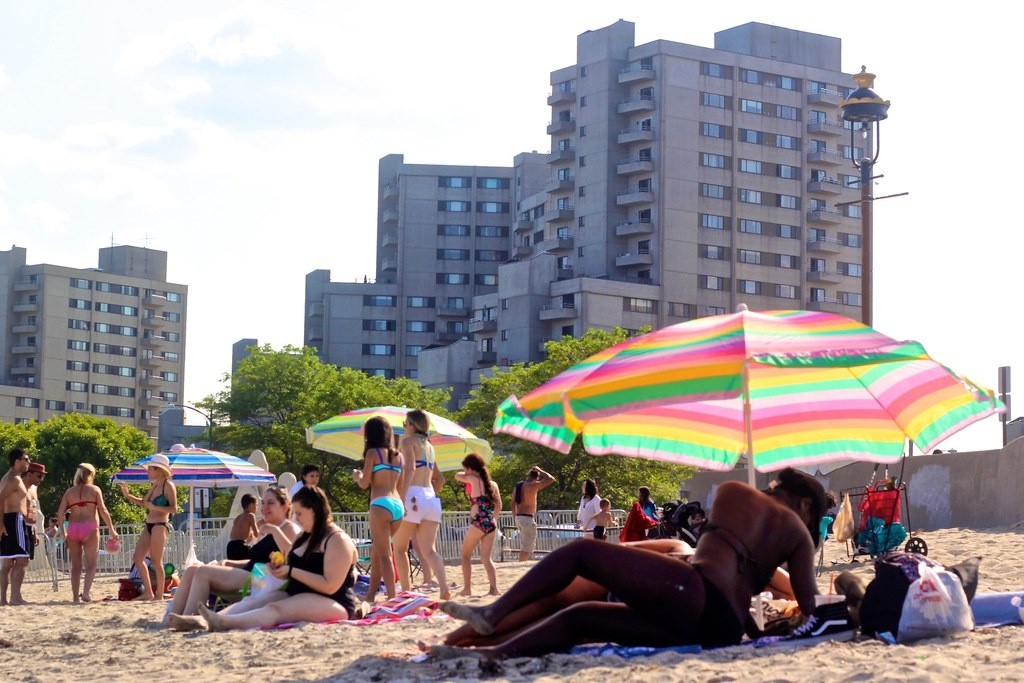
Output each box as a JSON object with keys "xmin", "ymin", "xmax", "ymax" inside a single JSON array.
[
  {"xmin": 27, "ymin": 462, "xmax": 47, "ymax": 475},
  {"xmin": 777, "ymin": 467, "xmax": 827, "ymax": 549},
  {"xmin": 139, "ymin": 454, "xmax": 174, "ymax": 479}
]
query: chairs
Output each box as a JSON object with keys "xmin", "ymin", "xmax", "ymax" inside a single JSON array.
[{"xmin": 353, "ymin": 535, "xmax": 424, "ymax": 583}]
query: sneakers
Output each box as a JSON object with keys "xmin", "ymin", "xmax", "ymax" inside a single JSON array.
[{"xmin": 775, "ymin": 600, "xmax": 857, "ymax": 646}]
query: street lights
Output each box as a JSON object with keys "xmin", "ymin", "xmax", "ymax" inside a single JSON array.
[
  {"xmin": 160, "ymin": 403, "xmax": 213, "ymax": 450},
  {"xmin": 834, "ymin": 65, "xmax": 909, "ymax": 325}
]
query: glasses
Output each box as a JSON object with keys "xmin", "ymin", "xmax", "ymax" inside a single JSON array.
[
  {"xmin": 32, "ymin": 471, "xmax": 45, "ymax": 479},
  {"xmin": 18, "ymin": 457, "xmax": 29, "ymax": 463}
]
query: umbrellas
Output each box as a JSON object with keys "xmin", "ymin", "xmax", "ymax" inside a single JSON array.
[
  {"xmin": 110, "ymin": 442, "xmax": 276, "ymax": 560},
  {"xmin": 495, "ymin": 304, "xmax": 1006, "ymax": 489},
  {"xmin": 307, "ymin": 406, "xmax": 493, "ymax": 473}
]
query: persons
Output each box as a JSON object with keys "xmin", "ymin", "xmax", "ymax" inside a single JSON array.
[
  {"xmin": 575, "ymin": 479, "xmax": 605, "ymax": 539},
  {"xmin": 118, "ymin": 454, "xmax": 177, "ymax": 602},
  {"xmin": 288, "ymin": 464, "xmax": 319, "ymax": 525},
  {"xmin": 0, "ymin": 449, "xmax": 48, "ymax": 605},
  {"xmin": 226, "ymin": 494, "xmax": 259, "ymax": 560},
  {"xmin": 44, "ymin": 509, "xmax": 70, "ymax": 571},
  {"xmin": 584, "ymin": 498, "xmax": 618, "ymax": 540},
  {"xmin": 167, "ymin": 485, "xmax": 358, "ymax": 632},
  {"xmin": 637, "ymin": 486, "xmax": 706, "ymax": 547},
  {"xmin": 353, "ymin": 416, "xmax": 405, "ymax": 603},
  {"xmin": 165, "ymin": 488, "xmax": 301, "ymax": 626},
  {"xmin": 58, "ymin": 463, "xmax": 119, "ymax": 602},
  {"xmin": 444, "ymin": 539, "xmax": 796, "ymax": 647},
  {"xmin": 511, "ymin": 466, "xmax": 555, "ymax": 562},
  {"xmin": 454, "ymin": 453, "xmax": 502, "ymax": 597},
  {"xmin": 394, "ymin": 410, "xmax": 451, "ymax": 601},
  {"xmin": 416, "ymin": 468, "xmax": 837, "ymax": 659}
]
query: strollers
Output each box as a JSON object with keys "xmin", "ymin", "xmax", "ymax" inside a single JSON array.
[{"xmin": 648, "ymin": 499, "xmax": 708, "ymax": 547}]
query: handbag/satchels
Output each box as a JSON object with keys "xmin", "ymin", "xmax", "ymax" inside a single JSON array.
[
  {"xmin": 896, "ymin": 564, "xmax": 975, "ymax": 643},
  {"xmin": 118, "ymin": 579, "xmax": 138, "ymax": 601},
  {"xmin": 832, "ymin": 492, "xmax": 855, "ymax": 542}
]
